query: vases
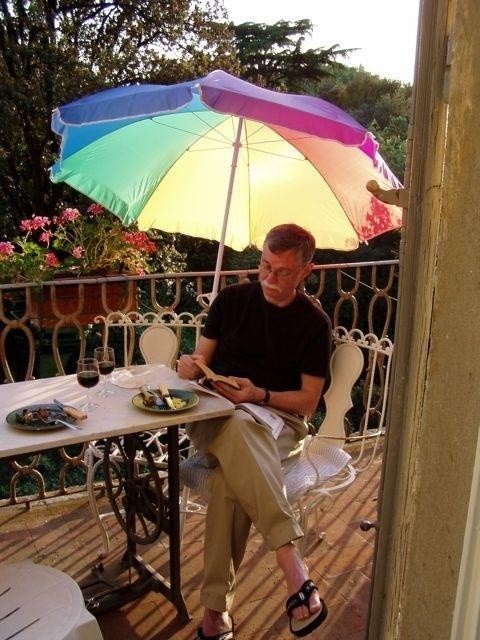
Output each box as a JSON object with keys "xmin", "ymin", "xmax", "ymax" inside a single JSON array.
[{"xmin": 26, "ymin": 276, "xmax": 139, "ymax": 326}]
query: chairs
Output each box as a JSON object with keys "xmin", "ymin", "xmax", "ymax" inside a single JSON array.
[
  {"xmin": 1, "ymin": 560, "xmax": 107, "ymax": 640},
  {"xmin": 89, "ymin": 311, "xmax": 216, "ymax": 555},
  {"xmin": 179, "ymin": 324, "xmax": 393, "ymax": 572}
]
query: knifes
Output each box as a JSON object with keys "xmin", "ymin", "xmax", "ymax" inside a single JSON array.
[
  {"xmin": 157, "ymin": 383, "xmax": 176, "ymax": 410},
  {"xmin": 54, "ymin": 399, "xmax": 87, "ymax": 421}
]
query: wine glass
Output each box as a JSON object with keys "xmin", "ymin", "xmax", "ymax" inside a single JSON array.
[{"xmin": 77, "ymin": 347, "xmax": 116, "ymax": 411}]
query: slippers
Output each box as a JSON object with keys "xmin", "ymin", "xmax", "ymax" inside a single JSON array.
[
  {"xmin": 284, "ymin": 580, "xmax": 327, "ymax": 636},
  {"xmin": 197, "ymin": 613, "xmax": 236, "ymax": 640}
]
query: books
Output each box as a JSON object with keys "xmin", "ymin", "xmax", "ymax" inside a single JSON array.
[
  {"xmin": 192, "ymin": 358, "xmax": 242, "ymax": 391},
  {"xmin": 198, "ymin": 382, "xmax": 285, "ymax": 441}
]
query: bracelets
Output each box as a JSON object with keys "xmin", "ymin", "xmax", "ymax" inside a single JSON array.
[{"xmin": 257, "ymin": 387, "xmax": 270, "ymax": 406}]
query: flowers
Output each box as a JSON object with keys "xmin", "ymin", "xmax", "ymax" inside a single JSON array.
[{"xmin": 0, "ymin": 203, "xmax": 155, "ymax": 275}]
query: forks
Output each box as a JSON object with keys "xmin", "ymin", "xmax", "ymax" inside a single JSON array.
[
  {"xmin": 148, "ymin": 389, "xmax": 167, "ymax": 410},
  {"xmin": 38, "ymin": 411, "xmax": 83, "ymax": 432}
]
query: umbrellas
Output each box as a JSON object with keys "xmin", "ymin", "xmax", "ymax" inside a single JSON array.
[{"xmin": 44, "ymin": 69, "xmax": 405, "ymax": 310}]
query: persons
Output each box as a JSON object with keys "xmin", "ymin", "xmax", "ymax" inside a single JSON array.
[{"xmin": 178, "ymin": 223, "xmax": 333, "ymax": 639}]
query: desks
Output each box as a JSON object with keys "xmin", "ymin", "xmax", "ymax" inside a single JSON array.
[{"xmin": 0, "ymin": 363, "xmax": 237, "ymax": 632}]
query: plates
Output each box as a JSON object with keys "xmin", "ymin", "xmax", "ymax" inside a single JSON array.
[
  {"xmin": 132, "ymin": 389, "xmax": 199, "ymax": 413},
  {"xmin": 6, "ymin": 404, "xmax": 78, "ymax": 431}
]
